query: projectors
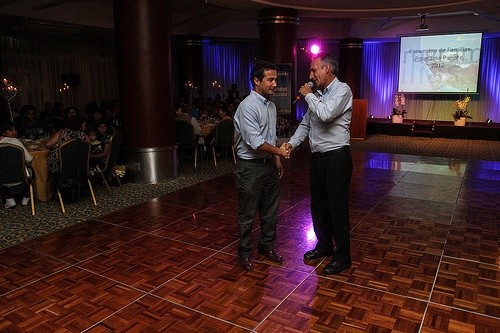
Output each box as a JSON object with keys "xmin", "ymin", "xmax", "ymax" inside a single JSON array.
[{"xmin": 416, "ymin": 25, "xmax": 429, "ymax": 31}]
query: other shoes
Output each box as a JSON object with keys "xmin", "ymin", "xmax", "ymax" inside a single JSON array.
[
  {"xmin": 5, "ymin": 198, "xmax": 16, "ymax": 208},
  {"xmin": 20, "ymin": 196, "xmax": 30, "ymax": 206}
]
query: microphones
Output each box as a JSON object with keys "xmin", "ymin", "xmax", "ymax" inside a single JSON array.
[{"xmin": 293, "ymin": 82, "xmax": 314, "ymax": 104}]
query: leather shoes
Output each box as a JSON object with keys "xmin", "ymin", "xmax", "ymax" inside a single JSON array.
[
  {"xmin": 322, "ymin": 260, "xmax": 351, "ymax": 273},
  {"xmin": 239, "ymin": 256, "xmax": 253, "ymax": 270},
  {"xmin": 257, "ymin": 248, "xmax": 282, "ymax": 261},
  {"xmin": 303, "ymin": 248, "xmax": 334, "ymax": 260}
]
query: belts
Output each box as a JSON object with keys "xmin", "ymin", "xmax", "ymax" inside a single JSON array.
[{"xmin": 240, "ymin": 156, "xmax": 274, "ymax": 163}]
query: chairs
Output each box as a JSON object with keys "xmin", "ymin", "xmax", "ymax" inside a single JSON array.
[
  {"xmin": 0, "ymin": 132, "xmax": 122, "ymax": 216},
  {"xmin": 176, "ymin": 118, "xmax": 236, "ymax": 168}
]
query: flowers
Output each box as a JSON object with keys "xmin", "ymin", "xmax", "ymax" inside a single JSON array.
[
  {"xmin": 389, "ymin": 94, "xmax": 407, "ymax": 118},
  {"xmin": 451, "ymin": 97, "xmax": 473, "ymax": 121}
]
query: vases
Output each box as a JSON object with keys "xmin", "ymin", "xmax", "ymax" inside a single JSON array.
[
  {"xmin": 392, "ymin": 114, "xmax": 403, "ymax": 124},
  {"xmin": 454, "ymin": 117, "xmax": 466, "ymax": 126}
]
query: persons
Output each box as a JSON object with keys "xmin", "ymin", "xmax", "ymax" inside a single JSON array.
[
  {"xmin": 233, "ymin": 61, "xmax": 292, "ymax": 271},
  {"xmin": 228, "ymin": 84, "xmax": 241, "ymax": 103},
  {"xmin": 175, "ymin": 94, "xmax": 237, "ymax": 164},
  {"xmin": 283, "ymin": 54, "xmax": 353, "ymax": 274},
  {"xmin": 0, "ymin": 122, "xmax": 33, "ymax": 209},
  {"xmin": 13, "ymin": 101, "xmax": 123, "ymax": 202}
]
